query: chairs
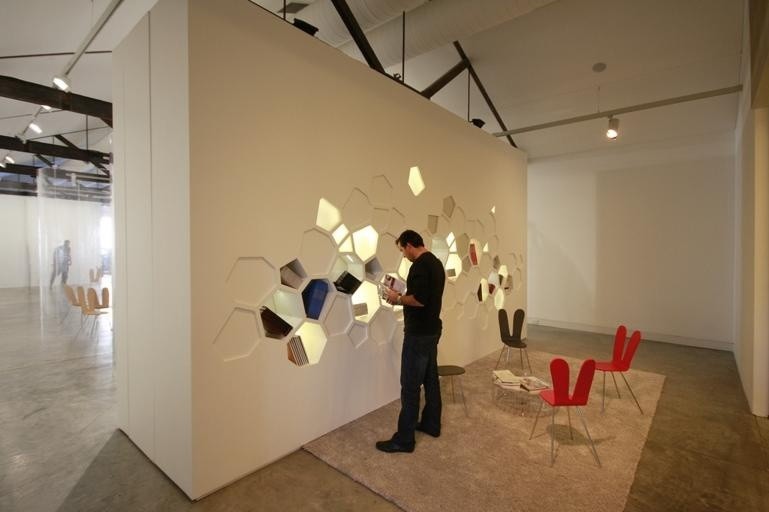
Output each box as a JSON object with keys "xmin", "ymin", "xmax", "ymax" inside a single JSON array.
[
  {"xmin": 438, "ymin": 308, "xmax": 644, "ymax": 469},
  {"xmin": 59, "ymin": 269, "xmax": 110, "ymax": 345}
]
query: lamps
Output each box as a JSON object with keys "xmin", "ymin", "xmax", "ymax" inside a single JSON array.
[
  {"xmin": 606, "ymin": 117, "xmax": 619, "ymax": 139},
  {"xmin": 0, "ymin": 72, "xmax": 71, "ymax": 169}
]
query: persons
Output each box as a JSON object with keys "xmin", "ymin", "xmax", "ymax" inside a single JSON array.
[
  {"xmin": 48, "ymin": 240, "xmax": 72, "ymax": 290},
  {"xmin": 374, "ymin": 229, "xmax": 446, "ymax": 454}
]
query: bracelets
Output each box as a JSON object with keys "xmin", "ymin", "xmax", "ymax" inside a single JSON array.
[{"xmin": 396, "ymin": 296, "xmax": 402, "ymax": 304}]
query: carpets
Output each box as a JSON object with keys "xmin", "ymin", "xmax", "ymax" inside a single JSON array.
[{"xmin": 301, "ymin": 347, "xmax": 666, "ymax": 512}]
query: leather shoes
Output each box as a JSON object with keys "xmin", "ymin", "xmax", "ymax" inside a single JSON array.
[
  {"xmin": 416, "ymin": 422, "xmax": 440, "ymax": 437},
  {"xmin": 375, "ymin": 439, "xmax": 416, "ymax": 452}
]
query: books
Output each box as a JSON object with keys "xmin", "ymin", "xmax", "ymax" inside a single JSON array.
[
  {"xmin": 493, "ymin": 369, "xmax": 521, "ymax": 386},
  {"xmin": 259, "ymin": 266, "xmax": 369, "ymax": 366},
  {"xmin": 519, "ymin": 375, "xmax": 548, "ymax": 392},
  {"xmin": 377, "ymin": 274, "xmax": 408, "ymax": 304}
]
